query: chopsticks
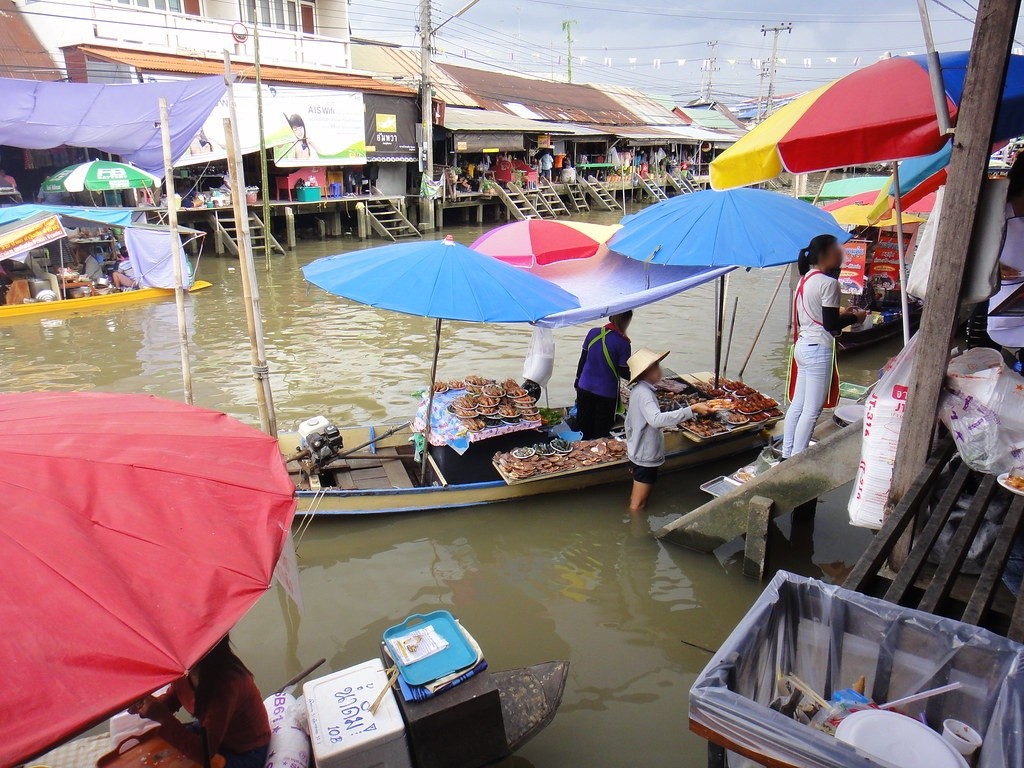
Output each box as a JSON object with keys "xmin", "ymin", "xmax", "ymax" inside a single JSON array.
[{"xmin": 786, "ymin": 672, "xmax": 832, "ymax": 709}]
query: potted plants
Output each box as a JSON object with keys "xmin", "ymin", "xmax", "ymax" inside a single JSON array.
[
  {"xmin": 510, "ymin": 179, "xmax": 522, "ymax": 193},
  {"xmin": 606, "ymin": 167, "xmax": 630, "ymax": 182},
  {"xmin": 481, "ymin": 179, "xmax": 494, "ymax": 194},
  {"xmin": 649, "ymin": 164, "xmax": 667, "ymax": 179}
]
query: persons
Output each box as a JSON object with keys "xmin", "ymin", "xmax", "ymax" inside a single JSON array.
[
  {"xmin": 580, "ymin": 151, "xmax": 588, "ymax": 179},
  {"xmin": 555, "ymin": 151, "xmax": 565, "ymax": 183},
  {"xmin": 0, "ymin": 168, "xmax": 17, "ymax": 186},
  {"xmin": 79, "ymin": 246, "xmax": 103, "ymax": 280},
  {"xmin": 38, "ymin": 172, "xmax": 70, "ymax": 204},
  {"xmin": 190, "ymin": 126, "xmax": 226, "ymax": 156},
  {"xmin": 114, "ymin": 247, "xmax": 137, "ymax": 291},
  {"xmin": 540, "ymin": 149, "xmax": 553, "ymax": 182},
  {"xmin": 127, "ymin": 633, "xmax": 271, "ymax": 768},
  {"xmin": 625, "ymin": 348, "xmax": 715, "ymax": 510},
  {"xmin": 291, "ymin": 113, "xmax": 318, "ymax": 159},
  {"xmin": 575, "ymin": 310, "xmax": 633, "ymax": 441},
  {"xmin": 780, "ymin": 235, "xmax": 866, "ymax": 463}
]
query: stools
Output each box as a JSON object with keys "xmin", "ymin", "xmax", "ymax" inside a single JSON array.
[{"xmin": 329, "ymin": 183, "xmax": 342, "ymax": 198}]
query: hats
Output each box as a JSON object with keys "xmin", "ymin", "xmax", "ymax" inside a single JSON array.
[{"xmin": 627, "ymin": 348, "xmax": 671, "ymax": 387}]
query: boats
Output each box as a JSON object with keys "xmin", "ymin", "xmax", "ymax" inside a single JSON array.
[
  {"xmin": 832, "ymin": 302, "xmax": 927, "ymax": 360},
  {"xmin": 276, "ymin": 369, "xmax": 782, "ymax": 520},
  {"xmin": 14, "ymin": 658, "xmax": 573, "ymax": 768},
  {"xmin": 0, "ymin": 279, "xmax": 216, "ymax": 319}
]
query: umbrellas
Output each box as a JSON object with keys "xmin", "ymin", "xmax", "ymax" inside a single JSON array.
[
  {"xmin": 0, "ymin": 391, "xmax": 298, "ymax": 768},
  {"xmin": 610, "ymin": 189, "xmax": 853, "ymax": 388},
  {"xmin": 301, "ymin": 235, "xmax": 581, "ymax": 484},
  {"xmin": 43, "ymin": 159, "xmax": 161, "ymax": 194},
  {"xmin": 708, "ymin": 50, "xmax": 1024, "ymax": 347},
  {"xmin": 468, "ymin": 215, "xmax": 623, "ymax": 268}
]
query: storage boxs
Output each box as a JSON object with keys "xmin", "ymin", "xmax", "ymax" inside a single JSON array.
[
  {"xmin": 296, "ymin": 186, "xmax": 321, "ymax": 202},
  {"xmin": 302, "ymin": 658, "xmax": 414, "ymax": 768},
  {"xmin": 379, "ymin": 639, "xmax": 508, "ymax": 768}
]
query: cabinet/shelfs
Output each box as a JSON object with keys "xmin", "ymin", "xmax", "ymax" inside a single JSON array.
[
  {"xmin": 0, "ymin": 275, "xmax": 31, "ymax": 306},
  {"xmin": 64, "ymin": 238, "xmax": 118, "ymax": 276}
]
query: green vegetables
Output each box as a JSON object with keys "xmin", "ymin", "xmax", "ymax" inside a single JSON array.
[{"xmin": 538, "ymin": 407, "xmax": 562, "ymax": 426}]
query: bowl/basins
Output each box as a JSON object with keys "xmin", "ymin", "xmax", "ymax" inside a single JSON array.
[
  {"xmin": 834, "ymin": 404, "xmax": 865, "ymax": 424},
  {"xmin": 96, "ymin": 278, "xmax": 109, "ymax": 286}
]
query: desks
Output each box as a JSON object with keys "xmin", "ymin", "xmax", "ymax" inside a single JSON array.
[
  {"xmin": 52, "ymin": 267, "xmax": 95, "ymax": 297},
  {"xmin": 174, "ymin": 174, "xmax": 226, "ymax": 193},
  {"xmin": 413, "ymin": 384, "xmax": 543, "ymax": 487}
]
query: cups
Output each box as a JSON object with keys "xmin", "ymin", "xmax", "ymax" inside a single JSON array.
[{"xmin": 943, "ymin": 719, "xmax": 982, "ymax": 756}]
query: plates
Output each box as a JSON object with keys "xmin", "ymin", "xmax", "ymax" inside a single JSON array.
[
  {"xmin": 834, "ymin": 710, "xmax": 971, "ymax": 768},
  {"xmin": 536, "ymin": 450, "xmax": 556, "ymax": 455},
  {"xmin": 509, "ymin": 447, "xmax": 534, "ymax": 459},
  {"xmin": 715, "ymin": 385, "xmax": 769, "ymax": 424},
  {"xmin": 550, "ymin": 440, "xmax": 573, "ymax": 452},
  {"xmin": 996, "ymin": 472, "xmax": 1024, "ymax": 497},
  {"xmin": 427, "ymin": 379, "xmax": 541, "ymax": 433}
]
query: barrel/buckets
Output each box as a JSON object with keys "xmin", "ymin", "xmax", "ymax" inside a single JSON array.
[
  {"xmin": 68, "ymin": 286, "xmax": 92, "ymax": 298},
  {"xmin": 95, "ymin": 253, "xmax": 104, "ymax": 263},
  {"xmin": 246, "ymin": 192, "xmax": 258, "ymax": 204},
  {"xmin": 113, "ymin": 227, "xmax": 121, "ymax": 235},
  {"xmin": 206, "ymin": 201, "xmax": 213, "ymax": 208}
]
